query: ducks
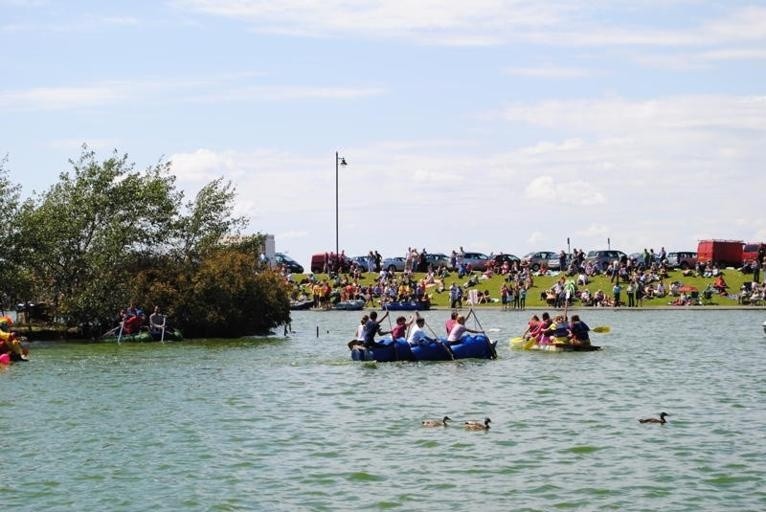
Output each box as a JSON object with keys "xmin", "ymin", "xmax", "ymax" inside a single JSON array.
[
  {"xmin": 463, "ymin": 418, "xmax": 491, "ymax": 431},
  {"xmin": 421, "ymin": 416, "xmax": 451, "ymax": 425},
  {"xmin": 638, "ymin": 412, "xmax": 670, "ymax": 423}
]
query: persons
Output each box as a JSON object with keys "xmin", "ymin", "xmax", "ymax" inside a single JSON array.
[
  {"xmin": 0, "ymin": 317, "xmax": 29, "ymax": 361},
  {"xmin": 271, "ymin": 246, "xmax": 766, "ymax": 347},
  {"xmin": 115, "ymin": 302, "xmax": 168, "ymax": 337}
]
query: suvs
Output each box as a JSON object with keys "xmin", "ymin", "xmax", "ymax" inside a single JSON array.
[{"xmin": 664, "ymin": 252, "xmax": 695, "ymax": 270}]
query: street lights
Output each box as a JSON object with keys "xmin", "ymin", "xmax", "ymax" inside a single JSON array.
[{"xmin": 333, "ymin": 156, "xmax": 348, "ymax": 272}]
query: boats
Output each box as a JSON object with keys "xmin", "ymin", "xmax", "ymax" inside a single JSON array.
[
  {"xmin": 348, "ymin": 332, "xmax": 497, "ymax": 365},
  {"xmin": 101, "ymin": 328, "xmax": 184, "ymax": 342},
  {"xmin": 0, "ymin": 341, "xmax": 34, "ymax": 362},
  {"xmin": 507, "ymin": 335, "xmax": 601, "ymax": 352}
]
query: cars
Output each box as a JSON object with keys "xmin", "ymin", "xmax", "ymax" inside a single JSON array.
[
  {"xmin": 351, "ymin": 256, "xmax": 383, "ymax": 271},
  {"xmin": 519, "ymin": 250, "xmax": 556, "ymax": 270},
  {"xmin": 425, "ymin": 254, "xmax": 449, "ymax": 269},
  {"xmin": 628, "ymin": 253, "xmax": 659, "ymax": 267},
  {"xmin": 15, "ymin": 302, "xmax": 35, "ymax": 314},
  {"xmin": 454, "ymin": 252, "xmax": 488, "ymax": 270},
  {"xmin": 378, "ymin": 256, "xmax": 406, "ymax": 272},
  {"xmin": 548, "ymin": 253, "xmax": 572, "ymax": 269},
  {"xmin": 489, "ymin": 254, "xmax": 520, "ymax": 271}
]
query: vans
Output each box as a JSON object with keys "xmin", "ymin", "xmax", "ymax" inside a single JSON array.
[
  {"xmin": 273, "ymin": 253, "xmax": 305, "ymax": 273},
  {"xmin": 585, "ymin": 250, "xmax": 627, "ymax": 269},
  {"xmin": 309, "ymin": 253, "xmax": 360, "ymax": 274}
]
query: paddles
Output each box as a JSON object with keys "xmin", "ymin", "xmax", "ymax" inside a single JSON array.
[
  {"xmin": 471, "ymin": 309, "xmax": 498, "ymax": 358},
  {"xmin": 524, "ymin": 332, "xmax": 539, "ymax": 349},
  {"xmin": 565, "ymin": 290, "xmax": 571, "ymax": 316},
  {"xmin": 590, "ymin": 327, "xmax": 611, "ymax": 333}
]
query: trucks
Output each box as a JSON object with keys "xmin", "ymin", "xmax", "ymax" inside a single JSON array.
[
  {"xmin": 740, "ymin": 242, "xmax": 765, "ymax": 265},
  {"xmin": 693, "ymin": 237, "xmax": 746, "ymax": 271}
]
